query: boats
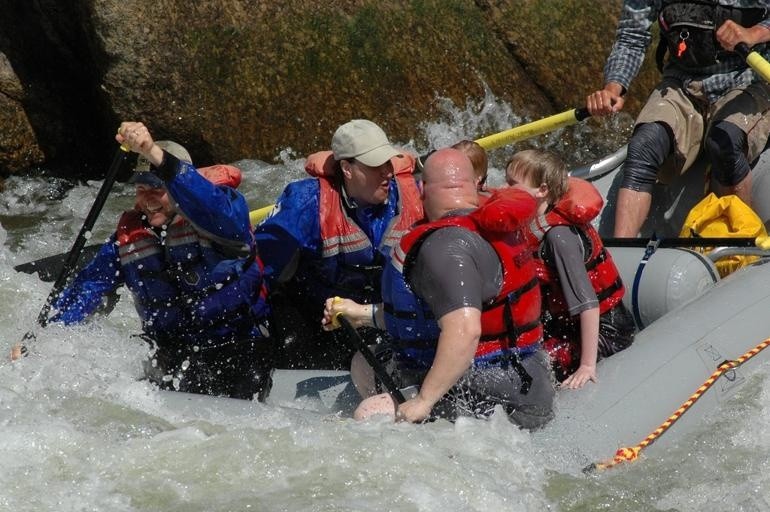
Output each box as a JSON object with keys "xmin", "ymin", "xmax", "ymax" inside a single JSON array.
[{"xmin": 134, "ymin": 128, "xmax": 768, "ymax": 485}]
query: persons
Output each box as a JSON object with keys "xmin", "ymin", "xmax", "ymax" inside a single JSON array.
[
  {"xmin": 8, "ymin": 118, "xmax": 638, "ymax": 429},
  {"xmin": 586, "ymin": 0, "xmax": 770, "ymax": 240}
]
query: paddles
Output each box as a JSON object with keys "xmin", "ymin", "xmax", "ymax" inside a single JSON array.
[{"xmin": 13, "ymin": 107, "xmax": 592, "ymax": 283}]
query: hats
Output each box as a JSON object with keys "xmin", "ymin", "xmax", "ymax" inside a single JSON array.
[
  {"xmin": 332, "ymin": 119, "xmax": 403, "ymax": 168},
  {"xmin": 129, "ymin": 141, "xmax": 191, "ymax": 183}
]
